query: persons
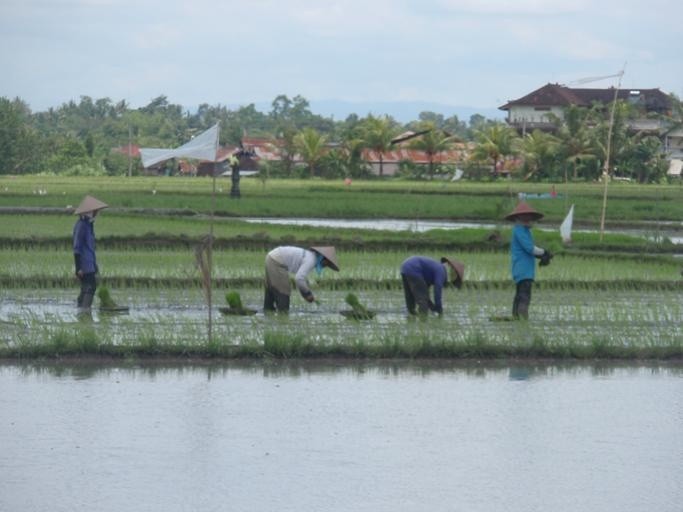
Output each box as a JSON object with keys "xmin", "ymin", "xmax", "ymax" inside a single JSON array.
[
  {"xmin": 230, "ymin": 162, "xmax": 242, "ymax": 199},
  {"xmin": 70, "ymin": 194, "xmax": 111, "ymax": 308},
  {"xmin": 262, "ymin": 245, "xmax": 340, "ymax": 316},
  {"xmin": 399, "ymin": 254, "xmax": 466, "ymax": 317},
  {"xmin": 502, "ymin": 199, "xmax": 554, "ymax": 321}
]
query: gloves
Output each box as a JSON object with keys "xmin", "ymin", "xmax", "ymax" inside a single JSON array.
[
  {"xmin": 428, "ymin": 302, "xmax": 442, "ymax": 313},
  {"xmin": 542, "ymin": 255, "xmax": 549, "ymax": 264}
]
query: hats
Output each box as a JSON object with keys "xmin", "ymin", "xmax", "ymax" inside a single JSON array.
[
  {"xmin": 73, "ymin": 194, "xmax": 107, "ymax": 216},
  {"xmin": 311, "ymin": 245, "xmax": 339, "ymax": 270},
  {"xmin": 233, "ymin": 147, "xmax": 242, "ymax": 156},
  {"xmin": 440, "ymin": 256, "xmax": 464, "ymax": 288},
  {"xmin": 504, "ymin": 202, "xmax": 543, "ymax": 221}
]
